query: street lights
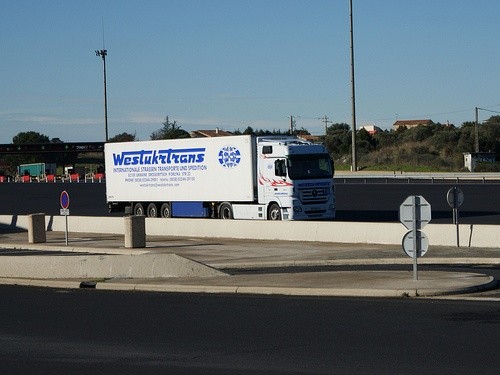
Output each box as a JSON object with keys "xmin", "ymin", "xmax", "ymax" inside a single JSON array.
[{"xmin": 95, "ymin": 49, "xmax": 108, "ymax": 143}]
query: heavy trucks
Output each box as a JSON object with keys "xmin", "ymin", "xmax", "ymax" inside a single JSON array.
[{"xmin": 104, "ymin": 134, "xmax": 337, "ymax": 220}]
